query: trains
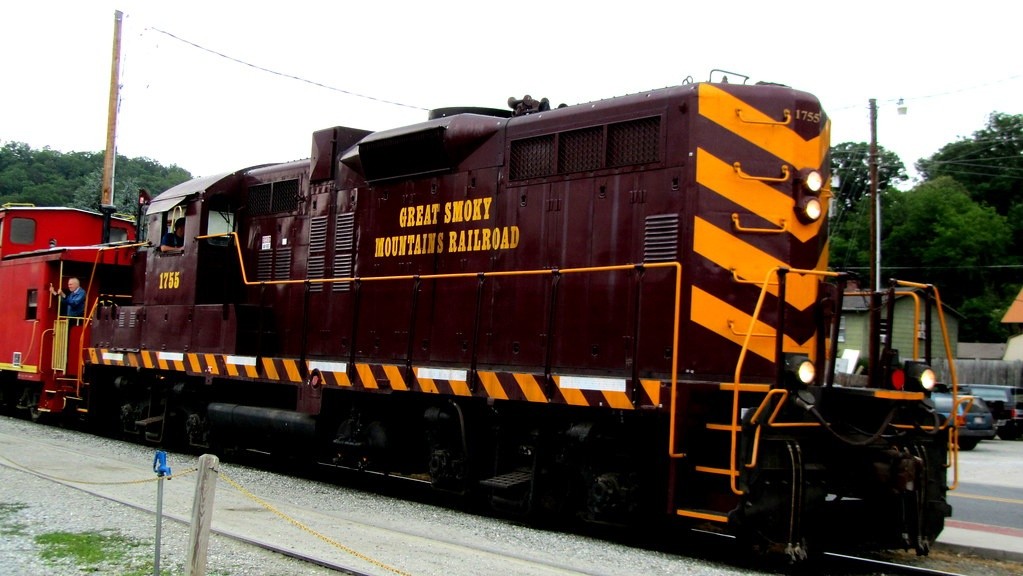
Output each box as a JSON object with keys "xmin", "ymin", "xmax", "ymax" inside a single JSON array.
[{"xmin": 0, "ymin": 63, "xmax": 976, "ymax": 572}]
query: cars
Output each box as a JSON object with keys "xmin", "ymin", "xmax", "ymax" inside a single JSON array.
[
  {"xmin": 931, "ymin": 391, "xmax": 998, "ymax": 452},
  {"xmin": 946, "ymin": 384, "xmax": 1023, "ymax": 442}
]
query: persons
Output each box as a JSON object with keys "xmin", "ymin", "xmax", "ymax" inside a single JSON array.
[
  {"xmin": 49, "ymin": 278, "xmax": 87, "ymax": 326},
  {"xmin": 159, "ymin": 217, "xmax": 186, "ymax": 252}
]
